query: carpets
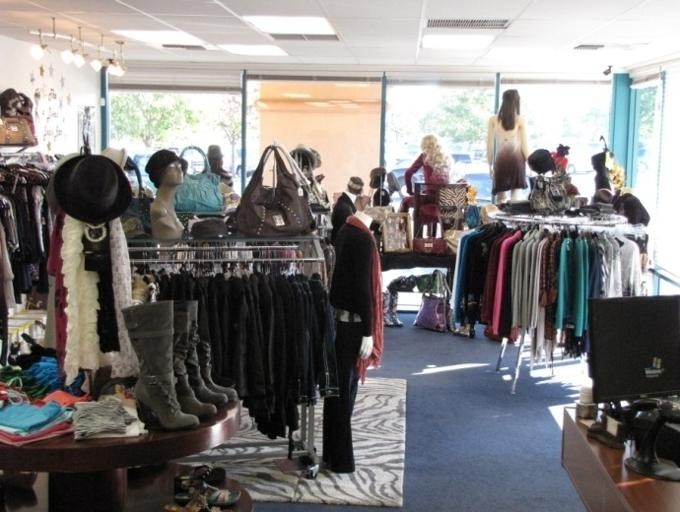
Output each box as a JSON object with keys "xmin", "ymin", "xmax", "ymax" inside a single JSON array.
[{"xmin": 167, "ymin": 378, "xmax": 409, "ymax": 509}]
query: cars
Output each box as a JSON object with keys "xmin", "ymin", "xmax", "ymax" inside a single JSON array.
[
  {"xmin": 386, "ymin": 143, "xmax": 498, "ymax": 205},
  {"xmin": 122, "ymin": 151, "xmax": 157, "ymax": 200},
  {"xmin": 565, "ymin": 148, "xmax": 596, "ymax": 175}
]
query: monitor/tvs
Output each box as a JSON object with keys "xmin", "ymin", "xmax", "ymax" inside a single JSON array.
[{"xmin": 587, "ymin": 295, "xmax": 680, "ymax": 411}]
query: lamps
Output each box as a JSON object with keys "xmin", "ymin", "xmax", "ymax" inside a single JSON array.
[{"xmin": 29, "ymin": 16, "xmax": 127, "ymax": 77}]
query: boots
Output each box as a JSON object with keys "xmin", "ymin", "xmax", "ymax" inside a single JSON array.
[
  {"xmin": 119, "ymin": 300, "xmax": 239, "ymax": 432},
  {"xmin": 376, "ymin": 290, "xmax": 405, "ymax": 329}
]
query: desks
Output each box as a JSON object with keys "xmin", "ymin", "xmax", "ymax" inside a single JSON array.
[
  {"xmin": 379, "ymin": 251, "xmax": 476, "ymax": 339},
  {"xmin": 559, "ymin": 406, "xmax": 680, "ymax": 512}
]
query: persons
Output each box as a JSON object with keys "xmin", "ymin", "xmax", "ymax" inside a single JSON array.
[
  {"xmin": 203, "ymin": 144, "xmax": 233, "ymax": 186},
  {"xmin": 145, "ymin": 148, "xmax": 189, "ymax": 241},
  {"xmin": 486, "ymin": 86, "xmax": 529, "ymax": 204},
  {"xmin": 323, "ymin": 208, "xmax": 377, "ymax": 477},
  {"xmin": 398, "ymin": 134, "xmax": 449, "ymax": 238}
]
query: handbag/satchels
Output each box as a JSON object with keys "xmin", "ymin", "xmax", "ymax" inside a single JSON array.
[
  {"xmin": 411, "ymin": 218, "xmax": 448, "ymax": 257},
  {"xmin": 527, "ymin": 168, "xmax": 571, "ymax": 215},
  {"xmin": 413, "ymin": 270, "xmax": 449, "ymax": 334},
  {"xmin": 168, "ymin": 146, "xmax": 226, "ymax": 213},
  {"xmin": 306, "ymin": 187, "xmax": 331, "ymax": 214},
  {"xmin": 114, "ymin": 158, "xmax": 156, "ymax": 240},
  {"xmin": 232, "ymin": 141, "xmax": 319, "ymax": 239},
  {"xmin": 0, "ymin": 115, "xmax": 40, "ymax": 147},
  {"xmin": 386, "ymin": 274, "xmax": 417, "ymax": 293}
]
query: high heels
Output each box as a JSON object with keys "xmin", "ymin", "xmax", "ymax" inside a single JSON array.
[{"xmin": 0, "ymin": 468, "xmax": 38, "ymax": 494}]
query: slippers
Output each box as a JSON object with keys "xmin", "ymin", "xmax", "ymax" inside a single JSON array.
[
  {"xmin": 162, "ymin": 497, "xmax": 233, "ymax": 512},
  {"xmin": 162, "ymin": 464, "xmax": 228, "ymax": 494},
  {"xmin": 173, "ymin": 478, "xmax": 243, "ymax": 507}
]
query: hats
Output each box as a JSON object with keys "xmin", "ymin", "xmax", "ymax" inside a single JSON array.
[
  {"xmin": 526, "ymin": 148, "xmax": 558, "ymax": 174},
  {"xmin": 145, "ymin": 149, "xmax": 188, "ymax": 189},
  {"xmin": 0, "ymin": 88, "xmax": 32, "ymax": 117},
  {"xmin": 289, "ymin": 142, "xmax": 322, "ymax": 172},
  {"xmin": 45, "ymin": 147, "xmax": 133, "ymax": 224},
  {"xmin": 345, "ymin": 168, "xmax": 390, "ymax": 206},
  {"xmin": 418, "ymin": 203, "xmax": 444, "ymax": 218},
  {"xmin": 190, "ymin": 217, "xmax": 230, "ymax": 241},
  {"xmin": 592, "ymin": 187, "xmax": 614, "ymax": 207}
]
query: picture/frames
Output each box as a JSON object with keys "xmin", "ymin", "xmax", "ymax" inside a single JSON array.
[{"xmin": 380, "ymin": 212, "xmax": 414, "ymax": 254}]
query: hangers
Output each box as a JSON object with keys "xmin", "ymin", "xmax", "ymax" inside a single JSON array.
[
  {"xmin": 128, "ymin": 239, "xmax": 319, "ymax": 280},
  {"xmin": 491, "ymin": 210, "xmax": 629, "ymax": 244}
]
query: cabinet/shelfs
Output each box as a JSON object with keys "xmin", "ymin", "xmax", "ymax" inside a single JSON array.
[{"xmin": 1, "ymin": 400, "xmax": 256, "ymax": 512}]
